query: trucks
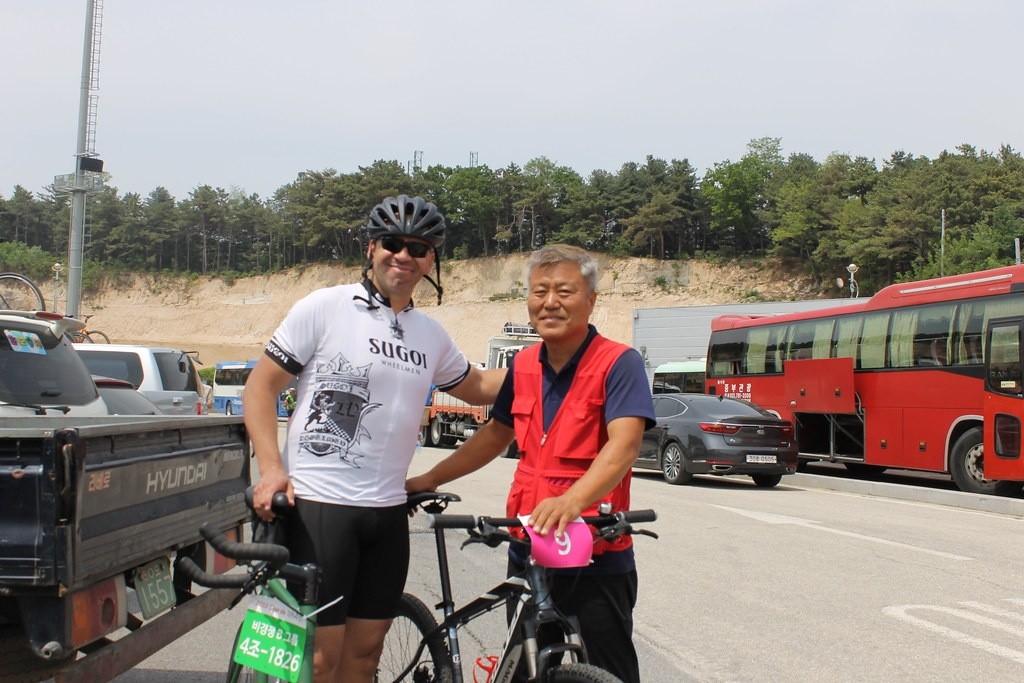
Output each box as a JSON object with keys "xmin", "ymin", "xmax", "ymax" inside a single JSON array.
[{"xmin": 0, "ymin": 412, "xmax": 267, "ymax": 683}]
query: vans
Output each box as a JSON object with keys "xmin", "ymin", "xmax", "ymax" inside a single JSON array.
[{"xmin": 72, "ymin": 343, "xmax": 203, "ymax": 417}]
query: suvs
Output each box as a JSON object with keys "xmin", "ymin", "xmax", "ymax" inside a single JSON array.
[{"xmin": 0, "ymin": 307, "xmax": 110, "ymax": 424}]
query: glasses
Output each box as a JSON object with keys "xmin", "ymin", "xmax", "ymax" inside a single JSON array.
[{"xmin": 371, "ymin": 236, "xmax": 435, "ymax": 259}]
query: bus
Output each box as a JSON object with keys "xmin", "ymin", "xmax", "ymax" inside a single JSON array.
[
  {"xmin": 649, "ymin": 358, "xmax": 710, "ymax": 394},
  {"xmin": 210, "ymin": 361, "xmax": 301, "ymax": 420},
  {"xmin": 703, "ymin": 264, "xmax": 1024, "ymax": 495}
]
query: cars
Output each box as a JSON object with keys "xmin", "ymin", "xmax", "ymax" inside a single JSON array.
[
  {"xmin": 629, "ymin": 391, "xmax": 800, "ymax": 490},
  {"xmin": 86, "ymin": 372, "xmax": 162, "ymax": 415}
]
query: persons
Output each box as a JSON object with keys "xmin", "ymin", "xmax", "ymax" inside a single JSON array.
[
  {"xmin": 406, "ymin": 243, "xmax": 658, "ymax": 680},
  {"xmin": 240, "ymin": 190, "xmax": 509, "ymax": 683}
]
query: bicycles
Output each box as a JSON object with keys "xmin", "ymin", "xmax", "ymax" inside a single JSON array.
[
  {"xmin": 177, "ymin": 486, "xmax": 325, "ymax": 682},
  {"xmin": 78, "ymin": 315, "xmax": 110, "ymax": 344},
  {"xmin": 0, "ymin": 274, "xmax": 46, "ymax": 313},
  {"xmin": 61, "ymin": 314, "xmax": 94, "ymax": 343},
  {"xmin": 371, "ymin": 489, "xmax": 657, "ymax": 683}
]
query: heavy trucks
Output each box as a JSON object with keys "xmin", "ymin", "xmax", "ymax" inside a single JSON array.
[{"xmin": 410, "ymin": 319, "xmax": 548, "ymax": 461}]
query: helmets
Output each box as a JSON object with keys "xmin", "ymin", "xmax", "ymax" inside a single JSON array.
[{"xmin": 366, "ymin": 195, "xmax": 447, "ymax": 250}]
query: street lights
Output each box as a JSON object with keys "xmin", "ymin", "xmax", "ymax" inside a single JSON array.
[
  {"xmin": 50, "ymin": 263, "xmax": 65, "ymax": 312},
  {"xmin": 835, "ymin": 264, "xmax": 860, "ymax": 298}
]
